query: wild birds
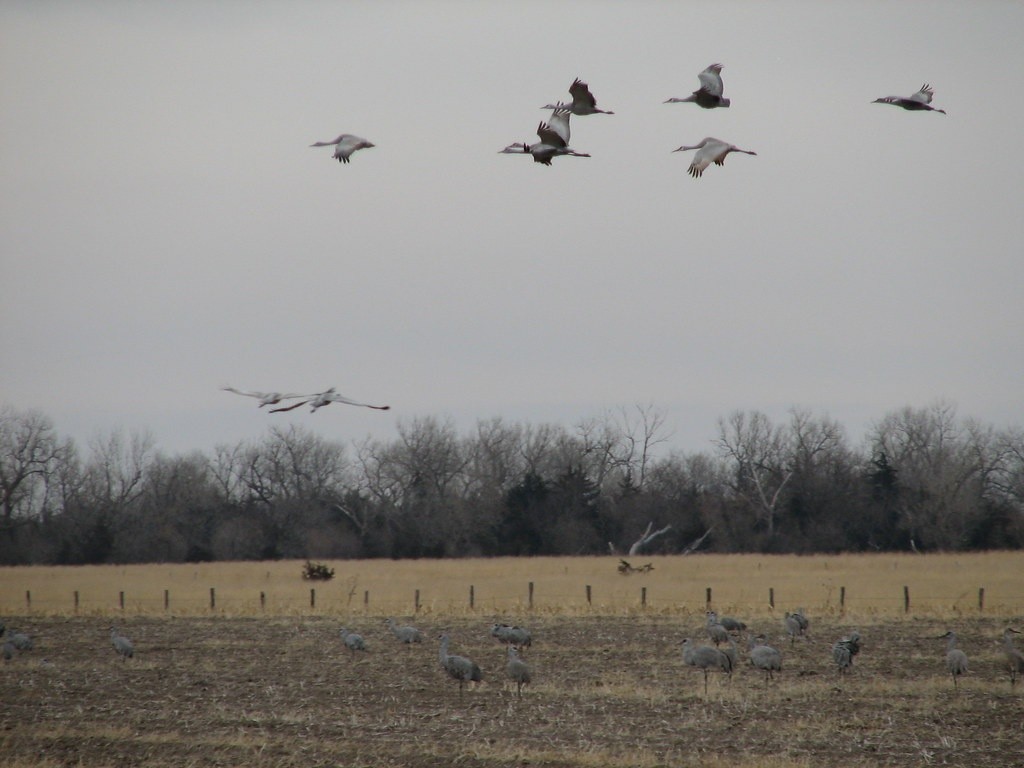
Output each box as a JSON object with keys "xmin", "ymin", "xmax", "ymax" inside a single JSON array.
[
  {"xmin": 924, "ymin": 629, "xmax": 977, "ymax": 692},
  {"xmin": 95, "ymin": 624, "xmax": 136, "ymax": 665},
  {"xmin": 221, "ymin": 386, "xmax": 390, "ymax": 414},
  {"xmin": 674, "ymin": 608, "xmax": 864, "ymax": 695},
  {"xmin": 496, "ymin": 76, "xmax": 615, "ymax": 167},
  {"xmin": 999, "ymin": 627, "xmax": 1024, "ymax": 686},
  {"xmin": 661, "ymin": 62, "xmax": 731, "ymax": 110},
  {"xmin": 309, "ymin": 132, "xmax": 376, "ymax": 164},
  {"xmin": 334, "ymin": 616, "xmax": 533, "ymax": 704},
  {"xmin": 869, "ymin": 83, "xmax": 948, "ymax": 115},
  {"xmin": 671, "ymin": 136, "xmax": 758, "ymax": 179}
]
